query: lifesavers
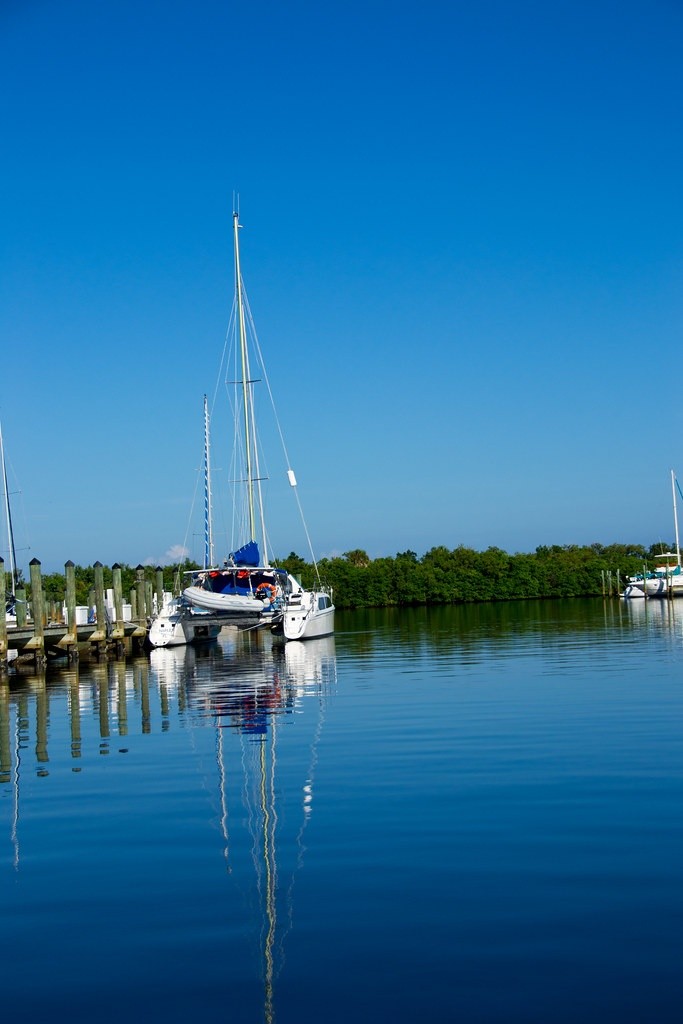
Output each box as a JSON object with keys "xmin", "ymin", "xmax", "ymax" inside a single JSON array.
[{"xmin": 255, "ymin": 582, "xmax": 276, "ymax": 603}]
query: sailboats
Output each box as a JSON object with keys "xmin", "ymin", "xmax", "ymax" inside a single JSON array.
[
  {"xmin": 147, "ymin": 636, "xmax": 343, "ymax": 1018},
  {"xmin": 625, "ymin": 470, "xmax": 683, "ymax": 599},
  {"xmin": 145, "ymin": 190, "xmax": 337, "ymax": 646}
]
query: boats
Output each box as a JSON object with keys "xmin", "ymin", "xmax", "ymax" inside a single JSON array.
[{"xmin": 182, "ymin": 586, "xmax": 272, "ymax": 613}]
